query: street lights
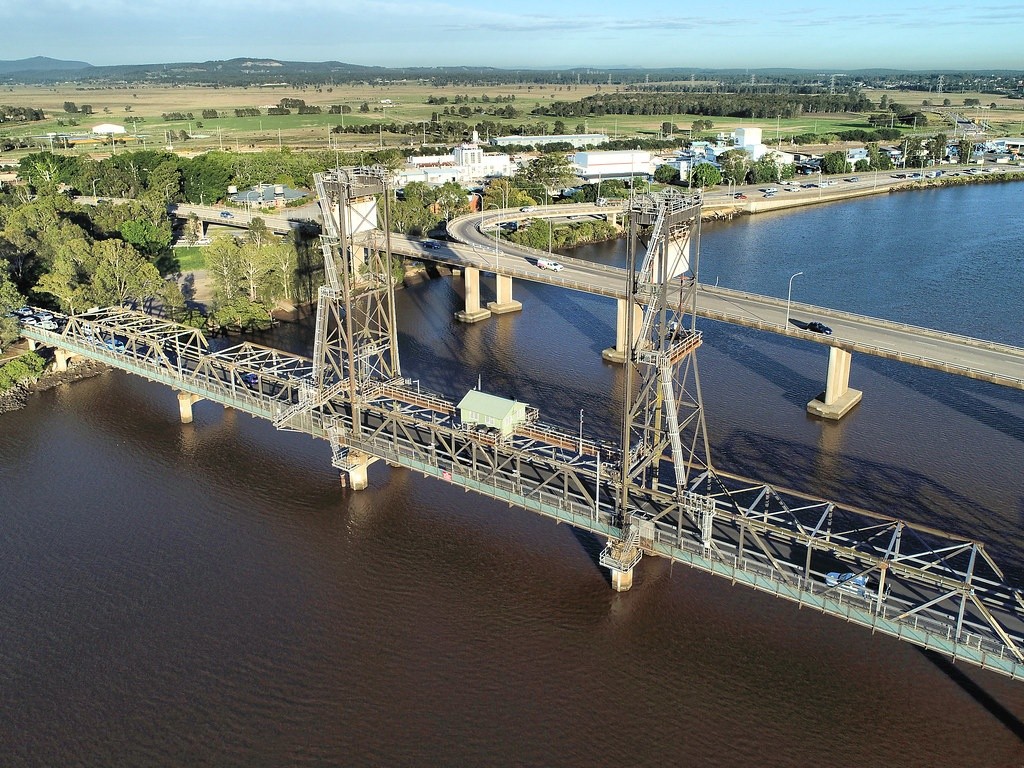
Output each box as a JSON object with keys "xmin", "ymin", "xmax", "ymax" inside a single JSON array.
[
  {"xmin": 471, "ymin": 181, "xmax": 510, "ymax": 268},
  {"xmin": 785, "ymin": 271, "xmax": 804, "ymax": 330}
]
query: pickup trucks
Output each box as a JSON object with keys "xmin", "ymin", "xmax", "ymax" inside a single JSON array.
[{"xmin": 826, "ymin": 572, "xmax": 891, "ymax": 605}]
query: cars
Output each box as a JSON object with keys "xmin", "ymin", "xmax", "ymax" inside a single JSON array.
[
  {"xmin": 423, "ymin": 241, "xmax": 441, "ymax": 250},
  {"xmin": 4, "ymin": 307, "xmax": 170, "ymax": 366},
  {"xmin": 239, "ymin": 373, "xmax": 259, "ymax": 384},
  {"xmin": 521, "ymin": 207, "xmax": 536, "ymax": 213},
  {"xmin": 687, "ymin": 155, "xmax": 1007, "ymax": 207},
  {"xmin": 806, "ymin": 322, "xmax": 833, "ymax": 336},
  {"xmin": 220, "ymin": 211, "xmax": 234, "ymax": 219}
]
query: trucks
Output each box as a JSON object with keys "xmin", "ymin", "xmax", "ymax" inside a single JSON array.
[{"xmin": 537, "ymin": 257, "xmax": 564, "ymax": 272}]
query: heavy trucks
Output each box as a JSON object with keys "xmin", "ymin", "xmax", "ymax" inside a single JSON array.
[
  {"xmin": 595, "ymin": 197, "xmax": 627, "ymax": 207},
  {"xmin": 274, "ymin": 186, "xmax": 285, "ymax": 202}
]
